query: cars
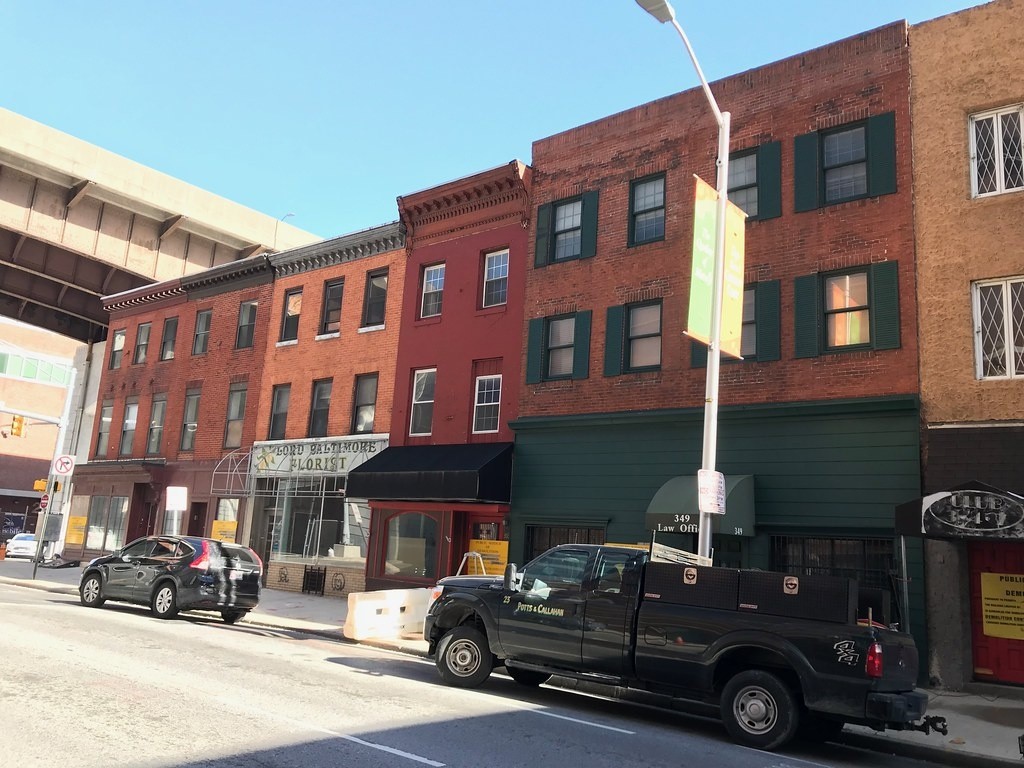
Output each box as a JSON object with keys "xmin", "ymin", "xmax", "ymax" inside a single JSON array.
[
  {"xmin": 5, "ymin": 531, "xmax": 41, "ymax": 560},
  {"xmin": 76, "ymin": 530, "xmax": 266, "ymax": 626}
]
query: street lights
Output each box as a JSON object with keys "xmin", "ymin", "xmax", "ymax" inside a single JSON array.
[{"xmin": 636, "ymin": 0, "xmax": 732, "ymax": 567}]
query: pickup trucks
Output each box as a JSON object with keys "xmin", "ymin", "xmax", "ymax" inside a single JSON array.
[{"xmin": 422, "ymin": 539, "xmax": 950, "ymax": 752}]
